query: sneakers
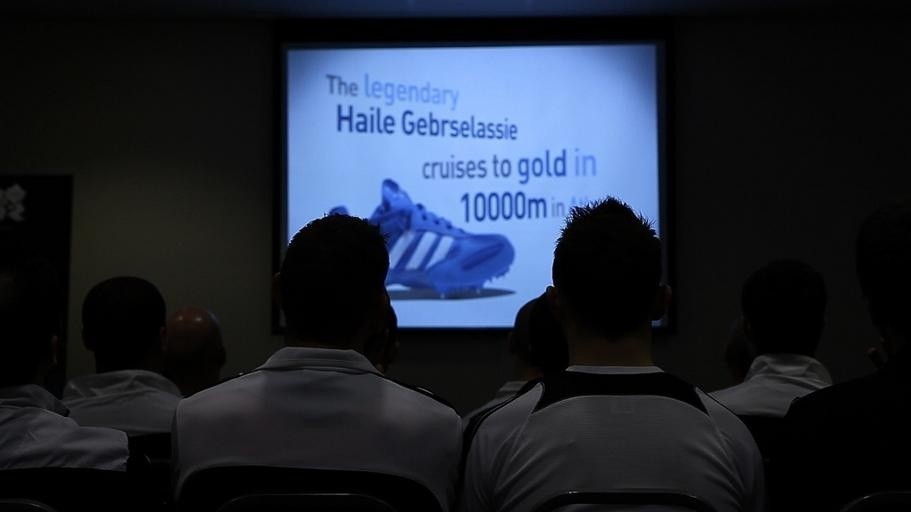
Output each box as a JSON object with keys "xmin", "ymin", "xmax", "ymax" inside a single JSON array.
[{"xmin": 328, "ymin": 179, "xmax": 514, "ymax": 297}]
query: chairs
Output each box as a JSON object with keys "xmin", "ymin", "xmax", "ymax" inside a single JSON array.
[
  {"xmin": 128, "ymin": 430, "xmax": 172, "ymax": 464},
  {"xmin": 0, "ymin": 467, "xmax": 152, "ymax": 512},
  {"xmin": 740, "ymin": 407, "xmax": 784, "ymax": 447},
  {"xmin": 535, "ymin": 486, "xmax": 712, "ymax": 512},
  {"xmin": 171, "ymin": 458, "xmax": 441, "ymax": 512}
]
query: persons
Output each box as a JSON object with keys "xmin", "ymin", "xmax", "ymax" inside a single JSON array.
[
  {"xmin": 59, "ymin": 276, "xmax": 184, "ymax": 437},
  {"xmin": 462, "ymin": 196, "xmax": 765, "ymax": 512},
  {"xmin": 175, "ymin": 214, "xmax": 464, "ymax": 512},
  {"xmin": 0, "ymin": 264, "xmax": 130, "ymax": 475},
  {"xmin": 167, "ymin": 306, "xmax": 228, "ymax": 399},
  {"xmin": 706, "ymin": 258, "xmax": 834, "ymax": 418},
  {"xmin": 464, "ymin": 298, "xmax": 550, "ymax": 426},
  {"xmin": 779, "ymin": 203, "xmax": 911, "ymax": 512}
]
